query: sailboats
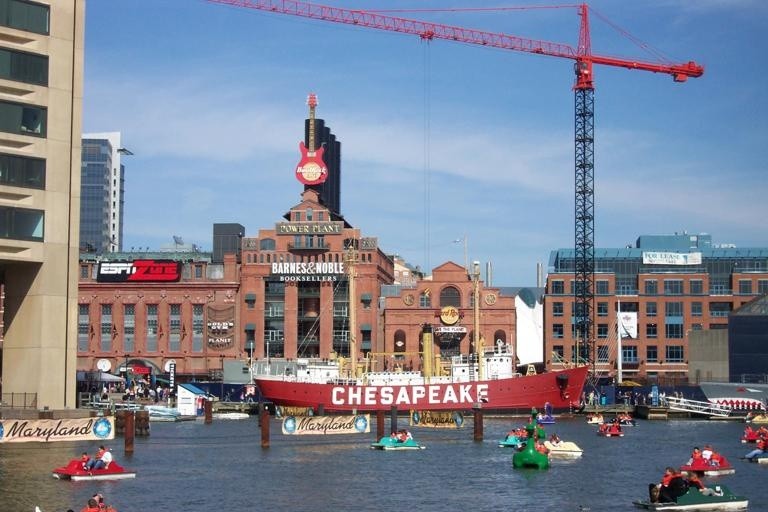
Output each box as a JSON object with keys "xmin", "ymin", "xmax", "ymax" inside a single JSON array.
[{"xmin": 250, "ymin": 242, "xmax": 592, "ymax": 417}]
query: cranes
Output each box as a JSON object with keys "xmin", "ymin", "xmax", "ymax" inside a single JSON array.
[{"xmin": 215, "ymin": 0, "xmax": 704, "ymax": 369}]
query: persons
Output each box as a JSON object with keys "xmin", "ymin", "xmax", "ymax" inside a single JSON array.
[
  {"xmin": 102, "ymin": 378, "xmax": 170, "ymax": 403},
  {"xmin": 82, "ymin": 446, "xmax": 112, "ymax": 476},
  {"xmin": 92, "ymin": 493, "xmax": 108, "ymax": 510},
  {"xmin": 82, "ymin": 499, "xmax": 101, "ymax": 512},
  {"xmin": 80, "ymin": 452, "xmax": 91, "ymax": 467},
  {"xmin": 648, "ymin": 465, "xmax": 684, "ymax": 503},
  {"xmin": 687, "ymin": 471, "xmax": 723, "ymax": 496},
  {"xmin": 396, "ymin": 432, "xmax": 407, "ymax": 443},
  {"xmin": 687, "ymin": 424, "xmax": 768, "ymax": 467},
  {"xmin": 390, "ymin": 431, "xmax": 395, "ymax": 439},
  {"xmin": 504, "ymin": 389, "xmax": 684, "ymax": 457},
  {"xmin": 396, "ymin": 431, "xmax": 403, "ymax": 440},
  {"xmin": 402, "ymin": 429, "xmax": 413, "ymax": 440}
]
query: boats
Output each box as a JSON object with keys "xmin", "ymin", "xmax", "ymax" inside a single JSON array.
[
  {"xmin": 736, "ymin": 407, "xmax": 767, "ymax": 468},
  {"xmin": 98, "ymin": 401, "xmax": 181, "ymax": 425},
  {"xmin": 682, "ymin": 463, "xmax": 736, "ymax": 481},
  {"xmin": 630, "ymin": 476, "xmax": 749, "ymax": 511},
  {"xmin": 52, "ymin": 457, "xmax": 135, "ymax": 482},
  {"xmin": 373, "ymin": 433, "xmax": 426, "ymax": 452},
  {"xmin": 501, "ymin": 403, "xmax": 638, "ymax": 470}
]
